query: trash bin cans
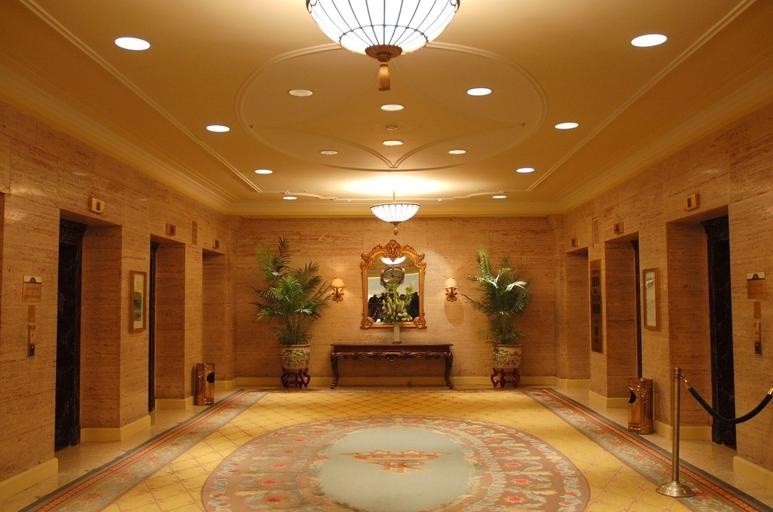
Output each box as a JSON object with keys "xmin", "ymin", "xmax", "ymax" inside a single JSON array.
[
  {"xmin": 626, "ymin": 378, "xmax": 654, "ymax": 436},
  {"xmin": 195, "ymin": 362, "xmax": 216, "ymax": 406}
]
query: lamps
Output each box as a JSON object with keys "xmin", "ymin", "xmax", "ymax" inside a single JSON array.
[
  {"xmin": 303, "ymin": 0, "xmax": 462, "ymax": 91},
  {"xmin": 444, "ymin": 278, "xmax": 458, "ymax": 302},
  {"xmin": 330, "ymin": 279, "xmax": 345, "ymax": 303},
  {"xmin": 369, "ymin": 190, "xmax": 420, "ymax": 235}
]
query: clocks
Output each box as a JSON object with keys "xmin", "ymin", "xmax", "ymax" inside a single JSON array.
[{"xmin": 380, "ymin": 266, "xmax": 405, "ymax": 289}]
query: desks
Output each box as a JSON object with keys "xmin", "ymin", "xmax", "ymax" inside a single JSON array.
[{"xmin": 329, "ymin": 342, "xmax": 455, "ymax": 391}]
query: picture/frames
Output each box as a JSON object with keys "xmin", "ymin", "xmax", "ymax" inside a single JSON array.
[
  {"xmin": 642, "ymin": 267, "xmax": 661, "ymax": 334},
  {"xmin": 128, "ymin": 269, "xmax": 147, "ymax": 334}
]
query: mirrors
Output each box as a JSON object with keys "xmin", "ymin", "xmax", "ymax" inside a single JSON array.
[{"xmin": 359, "ymin": 238, "xmax": 427, "ymax": 331}]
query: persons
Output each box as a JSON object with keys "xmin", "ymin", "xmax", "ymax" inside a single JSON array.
[{"xmin": 369, "ymin": 292, "xmax": 419, "ymax": 322}]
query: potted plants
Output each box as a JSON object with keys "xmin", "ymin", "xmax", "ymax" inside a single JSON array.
[
  {"xmin": 249, "ymin": 234, "xmax": 331, "ymax": 374},
  {"xmin": 379, "ymin": 284, "xmax": 413, "ymax": 344},
  {"xmin": 460, "ymin": 248, "xmax": 532, "ymax": 372}
]
